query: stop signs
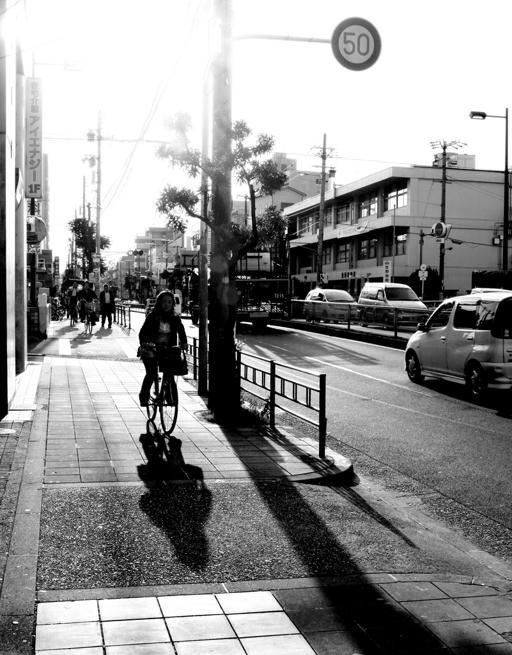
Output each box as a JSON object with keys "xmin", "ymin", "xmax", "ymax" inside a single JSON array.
[{"xmin": 434, "ymin": 223, "xmax": 443, "ymax": 236}]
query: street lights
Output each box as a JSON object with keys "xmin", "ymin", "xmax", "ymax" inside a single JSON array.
[
  {"xmin": 469, "ymin": 108, "xmax": 510, "ymax": 273},
  {"xmin": 117, "ymin": 269, "xmax": 153, "ymax": 306},
  {"xmin": 66, "ymin": 153, "xmax": 94, "ymax": 275},
  {"xmin": 85, "ymin": 121, "xmax": 107, "ymax": 294}
]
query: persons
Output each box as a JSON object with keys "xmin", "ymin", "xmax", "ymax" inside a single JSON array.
[
  {"xmin": 78, "ymin": 280, "xmax": 97, "ymax": 326},
  {"xmin": 99, "ymin": 283, "xmax": 116, "ymax": 329},
  {"xmin": 68, "ymin": 281, "xmax": 80, "ymax": 324},
  {"xmin": 138, "ymin": 288, "xmax": 188, "ymax": 408}
]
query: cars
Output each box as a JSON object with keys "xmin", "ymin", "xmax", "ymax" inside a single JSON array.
[
  {"xmin": 304, "ymin": 277, "xmax": 512, "ymax": 329},
  {"xmin": 403, "ymin": 290, "xmax": 512, "ymax": 397}
]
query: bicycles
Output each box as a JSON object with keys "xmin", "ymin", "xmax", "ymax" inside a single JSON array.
[
  {"xmin": 51, "ymin": 293, "xmax": 100, "ymax": 335},
  {"xmin": 138, "ymin": 342, "xmax": 186, "ymax": 435}
]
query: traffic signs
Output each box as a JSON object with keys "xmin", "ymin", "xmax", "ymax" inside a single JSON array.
[{"xmin": 333, "ymin": 16, "xmax": 382, "ymax": 70}]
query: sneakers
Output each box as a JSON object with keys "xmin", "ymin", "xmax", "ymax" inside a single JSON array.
[{"xmin": 139, "ymin": 391, "xmax": 150, "ymax": 406}]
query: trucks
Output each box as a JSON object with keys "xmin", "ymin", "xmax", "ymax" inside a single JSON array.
[{"xmin": 167, "ymin": 246, "xmax": 296, "ymax": 330}]
